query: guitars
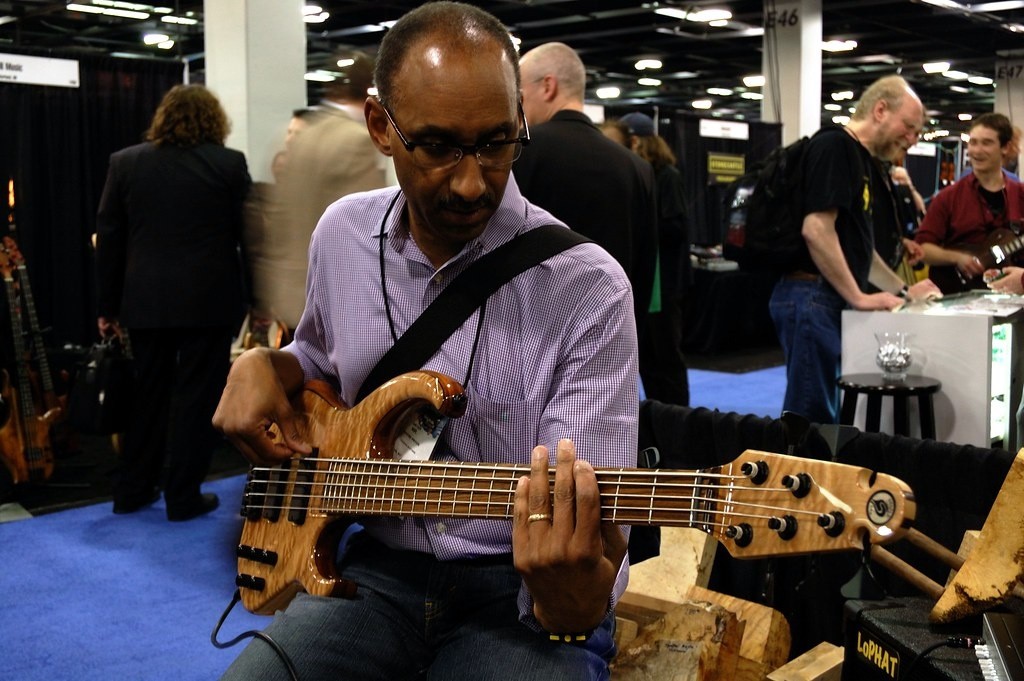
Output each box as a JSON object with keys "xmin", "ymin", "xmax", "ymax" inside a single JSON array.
[
  {"xmin": 236, "ymin": 366, "xmax": 918, "ymax": 623},
  {"xmin": 929, "ymin": 228, "xmax": 1024, "ymax": 296}
]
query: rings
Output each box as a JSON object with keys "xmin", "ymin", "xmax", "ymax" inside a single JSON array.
[{"xmin": 527, "ymin": 513, "xmax": 552, "ymax": 524}]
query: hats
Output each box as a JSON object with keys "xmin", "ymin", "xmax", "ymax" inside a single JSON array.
[{"xmin": 618, "ymin": 111, "xmax": 656, "ymax": 138}]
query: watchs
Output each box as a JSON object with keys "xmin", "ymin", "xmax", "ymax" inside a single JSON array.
[{"xmin": 530, "ymin": 597, "xmax": 610, "ymax": 642}]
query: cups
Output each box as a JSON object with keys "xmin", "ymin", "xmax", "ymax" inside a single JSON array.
[{"xmin": 876, "ymin": 333, "xmax": 912, "ymax": 379}]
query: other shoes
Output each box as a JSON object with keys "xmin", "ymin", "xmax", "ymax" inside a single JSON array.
[
  {"xmin": 169, "ymin": 492, "xmax": 219, "ymax": 522},
  {"xmin": 114, "ymin": 484, "xmax": 164, "ymax": 515}
]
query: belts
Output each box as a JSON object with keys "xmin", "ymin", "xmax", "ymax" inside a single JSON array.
[{"xmin": 786, "ymin": 272, "xmax": 824, "ymax": 284}]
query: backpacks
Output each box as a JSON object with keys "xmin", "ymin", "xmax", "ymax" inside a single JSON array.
[{"xmin": 720, "ymin": 125, "xmax": 849, "ymax": 283}]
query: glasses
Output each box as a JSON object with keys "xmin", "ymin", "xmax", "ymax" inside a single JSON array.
[{"xmin": 379, "ymin": 99, "xmax": 531, "ymax": 170}]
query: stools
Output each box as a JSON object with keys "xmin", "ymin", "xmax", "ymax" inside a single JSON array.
[{"xmin": 836, "ymin": 373, "xmax": 941, "ymax": 441}]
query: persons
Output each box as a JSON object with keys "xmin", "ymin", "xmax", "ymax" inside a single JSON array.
[
  {"xmin": 915, "ymin": 112, "xmax": 1024, "ymax": 296},
  {"xmin": 97, "ymin": 84, "xmax": 254, "ymax": 520},
  {"xmin": 274, "ymin": 58, "xmax": 388, "ymax": 331},
  {"xmin": 214, "ymin": 2, "xmax": 637, "ymax": 681},
  {"xmin": 721, "ymin": 164, "xmax": 764, "ymax": 260},
  {"xmin": 766, "ymin": 75, "xmax": 927, "ymax": 421},
  {"xmin": 513, "ymin": 40, "xmax": 658, "ymax": 325},
  {"xmin": 609, "ymin": 111, "xmax": 693, "ymax": 399}
]
